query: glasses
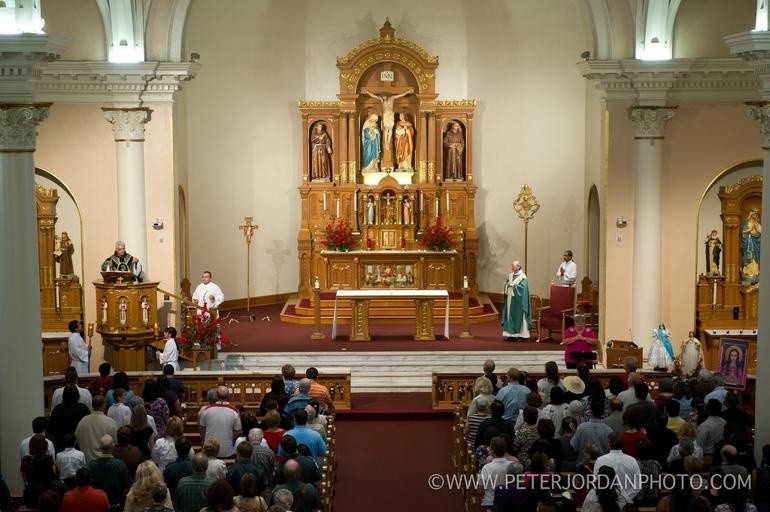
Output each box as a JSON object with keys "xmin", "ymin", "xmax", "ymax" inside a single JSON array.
[{"xmin": 116, "ymin": 247, "xmax": 126, "ymax": 250}]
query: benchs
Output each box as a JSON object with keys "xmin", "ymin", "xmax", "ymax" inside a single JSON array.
[
  {"xmin": 182, "ymin": 417, "xmax": 338, "ymax": 512},
  {"xmin": 449, "ymin": 405, "xmax": 482, "ymax": 511}
]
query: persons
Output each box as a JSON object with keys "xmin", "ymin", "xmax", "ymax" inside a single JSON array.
[
  {"xmin": 297, "ymin": 444, "xmax": 312, "ymax": 457},
  {"xmin": 56, "ymin": 434, "xmax": 86, "ymax": 490},
  {"xmin": 269, "ymin": 489, "xmax": 294, "ymax": 512},
  {"xmin": 622, "ymin": 383, "xmax": 656, "ymax": 431},
  {"xmin": 274, "ymin": 435, "xmax": 322, "ymax": 491},
  {"xmin": 129, "ymin": 404, "xmax": 155, "ymax": 459},
  {"xmin": 156, "ymin": 375, "xmax": 185, "ymax": 423},
  {"xmin": 723, "ymin": 347, "xmax": 743, "ymax": 384},
  {"xmin": 478, "ymin": 438, "xmax": 514, "ymax": 512},
  {"xmin": 619, "ymin": 356, "xmax": 637, "ymax": 391},
  {"xmin": 617, "ymin": 372, "xmax": 652, "ymax": 412},
  {"xmin": 671, "ymin": 381, "xmax": 694, "ymax": 419},
  {"xmin": 284, "ymin": 378, "xmax": 312, "ymax": 414},
  {"xmin": 656, "ymin": 478, "xmax": 712, "ymax": 512},
  {"xmin": 21, "ymin": 434, "xmax": 56, "ymax": 509},
  {"xmin": 575, "ymin": 443, "xmax": 604, "ymax": 511},
  {"xmin": 514, "ymin": 407, "xmax": 541, "ymax": 465},
  {"xmin": 689, "ymin": 398, "xmax": 708, "ymax": 422},
  {"xmin": 670, "ymin": 439, "xmax": 694, "ymax": 473},
  {"xmin": 721, "ymin": 391, "xmax": 754, "ymax": 474},
  {"xmin": 501, "ymin": 260, "xmax": 532, "ymax": 342},
  {"xmin": 282, "ymin": 410, "xmax": 327, "ymax": 461},
  {"xmin": 704, "ymin": 371, "xmax": 730, "ymax": 412},
  {"xmin": 570, "ymin": 398, "xmax": 616, "ymax": 480},
  {"xmin": 304, "ymin": 404, "xmax": 327, "ymax": 440},
  {"xmin": 681, "ymin": 330, "xmax": 704, "ymax": 377},
  {"xmin": 367, "ymin": 198, "xmax": 374, "ymax": 225},
  {"xmin": 515, "ymin": 393, "xmax": 544, "ymax": 436},
  {"xmin": 594, "ymin": 436, "xmax": 642, "ymax": 512},
  {"xmin": 141, "ymin": 481, "xmax": 174, "ymax": 512},
  {"xmin": 581, "ymin": 466, "xmax": 621, "ymax": 512},
  {"xmin": 162, "ymin": 437, "xmax": 192, "ymax": 503},
  {"xmin": 604, "ymin": 376, "xmax": 626, "ymax": 398},
  {"xmin": 294, "ymin": 366, "xmax": 333, "ymax": 415},
  {"xmin": 648, "ymin": 413, "xmax": 679, "ymax": 452},
  {"xmin": 366, "ymin": 265, "xmax": 412, "ymax": 287},
  {"xmin": 719, "ymin": 445, "xmax": 748, "ymax": 480},
  {"xmin": 603, "ymin": 399, "xmax": 626, "ymax": 450},
  {"xmin": 192, "ymin": 271, "xmax": 224, "ymax": 351},
  {"xmin": 265, "ymin": 398, "xmax": 279, "ymax": 411},
  {"xmin": 151, "ymin": 416, "xmax": 195, "ymax": 475},
  {"xmin": 260, "ymin": 377, "xmax": 291, "ymax": 415},
  {"xmin": 61, "ymin": 467, "xmax": 111, "ymax": 512},
  {"xmin": 272, "ymin": 458, "xmax": 322, "ymax": 512},
  {"xmin": 393, "ymin": 112, "xmax": 415, "ymax": 171},
  {"xmin": 129, "ymin": 395, "xmax": 158, "ymax": 438},
  {"xmin": 363, "ymin": 88, "xmax": 414, "ymax": 152},
  {"xmin": 467, "ymin": 377, "xmax": 497, "ymax": 421},
  {"xmin": 156, "ymin": 327, "xmax": 180, "ymax": 372},
  {"xmin": 235, "ymin": 404, "xmax": 245, "ymax": 413},
  {"xmin": 655, "ymin": 378, "xmax": 675, "ymax": 411},
  {"xmin": 476, "ymin": 359, "xmax": 503, "ymax": 387},
  {"xmin": 562, "ymin": 314, "xmax": 599, "ymax": 369},
  {"xmin": 36, "ymin": 489, "xmax": 60, "ymax": 512},
  {"xmin": 228, "ymin": 441, "xmax": 266, "ymax": 496},
  {"xmin": 59, "ymin": 232, "xmax": 75, "ymax": 276},
  {"xmin": 704, "ymin": 229, "xmax": 722, "ymax": 276},
  {"xmin": 527, "ymin": 380, "xmax": 537, "ymax": 392},
  {"xmin": 752, "ymin": 444, "xmax": 770, "ymax": 512},
  {"xmin": 555, "ymin": 250, "xmax": 577, "ymax": 284},
  {"xmin": 684, "ymin": 455, "xmax": 708, "ymax": 496},
  {"xmin": 543, "ymin": 386, "xmax": 570, "ymax": 459},
  {"xmin": 715, "ymin": 480, "xmax": 758, "ymax": 512},
  {"xmin": 101, "ymin": 241, "xmax": 142, "ymax": 281},
  {"xmin": 443, "ymin": 121, "xmax": 464, "ymax": 179},
  {"xmin": 647, "ymin": 323, "xmax": 675, "ymax": 371},
  {"xmin": 665, "ymin": 400, "xmax": 686, "ymax": 439},
  {"xmin": 496, "ymin": 368, "xmax": 532, "ymax": 427},
  {"xmin": 580, "ymin": 378, "xmax": 609, "ymax": 422},
  {"xmin": 49, "ymin": 383, "xmax": 91, "ymax": 448},
  {"xmin": 123, "ymin": 460, "xmax": 174, "ymax": 512},
  {"xmin": 741, "ymin": 207, "xmax": 762, "ymax": 283},
  {"xmin": 199, "ymin": 386, "xmax": 242, "ymax": 459},
  {"xmin": 529, "ymin": 419, "xmax": 557, "ymax": 459},
  {"xmin": 73, "ymin": 395, "xmax": 119, "ymax": 465},
  {"xmin": 310, "ymin": 401, "xmax": 327, "ymax": 429},
  {"xmin": 520, "ymin": 452, "xmax": 552, "ymax": 493},
  {"xmin": 537, "ymin": 361, "xmax": 565, "ymax": 405},
  {"xmin": 577, "ymin": 364, "xmax": 590, "ymax": 385},
  {"xmin": 563, "ymin": 374, "xmax": 585, "ymax": 404},
  {"xmin": 233, "ymin": 472, "xmax": 269, "ymax": 512},
  {"xmin": 199, "ymin": 479, "xmax": 240, "ymax": 512},
  {"xmin": 403, "ymin": 198, "xmax": 410, "ymax": 224},
  {"xmin": 103, "ymin": 372, "xmax": 134, "ymax": 416},
  {"xmin": 618, "ymin": 411, "xmax": 647, "ymax": 460},
  {"xmin": 163, "ymin": 364, "xmax": 185, "ymax": 406},
  {"xmin": 700, "ymin": 468, "xmax": 728, "ymax": 512},
  {"xmin": 88, "ymin": 434, "xmax": 132, "ymax": 512},
  {"xmin": 536, "ymin": 474, "xmax": 577, "ymax": 512},
  {"xmin": 361, "ymin": 114, "xmax": 382, "ymax": 170},
  {"xmin": 50, "ymin": 367, "xmax": 92, "ymax": 413},
  {"xmin": 666, "ymin": 422, "xmax": 704, "ymax": 470},
  {"xmin": 697, "ymin": 399, "xmax": 727, "ymax": 467},
  {"xmin": 20, "ymin": 416, "xmax": 56, "ymax": 483},
  {"xmin": 493, "ymin": 462, "xmax": 528, "ymax": 512},
  {"xmin": 246, "ymin": 427, "xmax": 274, "ymax": 472},
  {"xmin": 113, "ymin": 426, "xmax": 145, "ymax": 480},
  {"xmin": 176, "ymin": 452, "xmax": 217, "ymax": 512},
  {"xmin": 197, "ymin": 388, "xmax": 219, "ymax": 415},
  {"xmin": 485, "ymin": 434, "xmax": 519, "ymax": 465},
  {"xmin": 623, "ymin": 502, "xmax": 640, "ymax": 512},
  {"xmin": 281, "ymin": 364, "xmax": 300, "ymax": 396},
  {"xmin": 464, "ymin": 398, "xmax": 492, "ymax": 453},
  {"xmin": 474, "ymin": 400, "xmax": 515, "ymax": 453},
  {"xmin": 140, "ymin": 379, "xmax": 170, "ymax": 442},
  {"xmin": 635, "ymin": 439, "xmax": 663, "ymax": 508},
  {"xmin": 85, "ymin": 363, "xmax": 113, "ymax": 397},
  {"xmin": 68, "ymin": 320, "xmax": 92, "ymax": 374},
  {"xmin": 311, "ymin": 123, "xmax": 333, "ymax": 180},
  {"xmin": 107, "ymin": 388, "xmax": 132, "ymax": 428},
  {"xmin": 559, "ymin": 416, "xmax": 579, "ymax": 473},
  {"xmin": 203, "ymin": 438, "xmax": 229, "ymax": 480},
  {"xmin": 262, "ymin": 410, "xmax": 288, "ymax": 458},
  {"xmin": 234, "ymin": 415, "xmax": 269, "ymax": 450}
]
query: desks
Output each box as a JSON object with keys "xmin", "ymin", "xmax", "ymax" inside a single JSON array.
[{"xmin": 336, "ymin": 289, "xmax": 448, "ymax": 341}]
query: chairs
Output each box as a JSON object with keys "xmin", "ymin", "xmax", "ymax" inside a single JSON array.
[{"xmin": 536, "ymin": 279, "xmax": 577, "ymax": 347}]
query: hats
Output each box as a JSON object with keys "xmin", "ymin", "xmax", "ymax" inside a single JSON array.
[
  {"xmin": 562, "ymin": 376, "xmax": 585, "ymax": 394},
  {"xmin": 476, "ymin": 397, "xmax": 490, "ymax": 410}
]
